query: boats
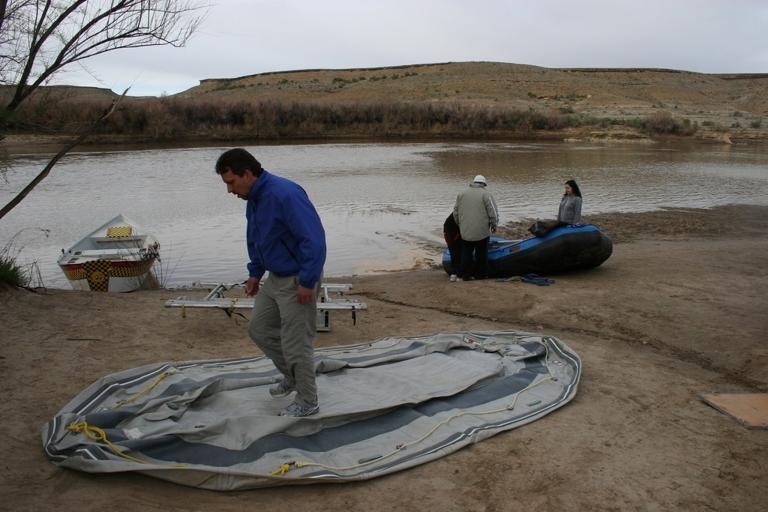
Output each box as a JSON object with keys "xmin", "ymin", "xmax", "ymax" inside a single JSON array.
[
  {"xmin": 438, "ymin": 222, "xmax": 616, "ymax": 277},
  {"xmin": 55, "ymin": 210, "xmax": 162, "ymax": 295}
]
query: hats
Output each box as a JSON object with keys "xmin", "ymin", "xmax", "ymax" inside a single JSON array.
[{"xmin": 473, "ymin": 174, "xmax": 487, "ymax": 186}]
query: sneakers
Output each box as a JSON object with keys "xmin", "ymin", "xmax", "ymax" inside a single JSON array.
[
  {"xmin": 457, "ymin": 276, "xmax": 488, "ymax": 282},
  {"xmin": 268, "ymin": 378, "xmax": 297, "ymax": 399},
  {"xmin": 277, "ymin": 401, "xmax": 320, "ymax": 420},
  {"xmin": 450, "ymin": 274, "xmax": 456, "ymax": 281}
]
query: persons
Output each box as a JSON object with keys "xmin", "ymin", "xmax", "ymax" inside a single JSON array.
[
  {"xmin": 557, "ymin": 179, "xmax": 583, "ymax": 228},
  {"xmin": 443, "ymin": 212, "xmax": 464, "ymax": 282},
  {"xmin": 452, "ymin": 174, "xmax": 499, "ymax": 281},
  {"xmin": 216, "ymin": 148, "xmax": 327, "ymax": 418}
]
